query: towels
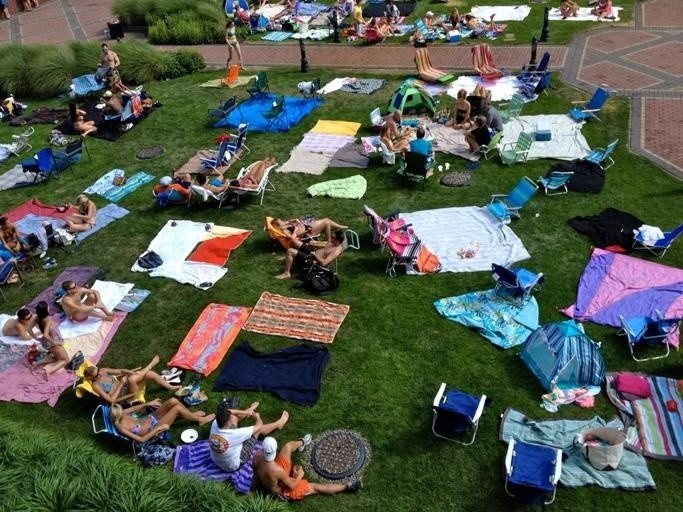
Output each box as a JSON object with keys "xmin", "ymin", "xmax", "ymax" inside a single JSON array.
[
  {"xmin": 224, "ymin": 1, "xmax": 626, "ymax": 50},
  {"xmin": 0, "ymin": 73, "xmax": 155, "ymax": 411},
  {"xmin": 124, "ymin": 288, "xmax": 353, "ymax": 500}
]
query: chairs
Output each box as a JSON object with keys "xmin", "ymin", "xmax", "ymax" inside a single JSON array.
[
  {"xmin": 363, "ymin": 208, "xmax": 399, "ymax": 245},
  {"xmin": 615, "ymin": 308, "xmax": 683, "ymax": 363},
  {"xmin": 237, "ymin": 160, "xmax": 279, "ymax": 193},
  {"xmin": 487, "ymin": 176, "xmax": 540, "ymax": 224},
  {"xmin": 632, "ymin": 225, "xmax": 682, "ymax": 260},
  {"xmin": 215, "ymin": 123, "xmax": 251, "ymax": 161},
  {"xmin": 372, "ymin": 139, "xmax": 408, "ymax": 165},
  {"xmin": 299, "ymin": 77, "xmax": 320, "ymax": 105},
  {"xmin": 520, "ymin": 72, "xmax": 550, "ymax": 104},
  {"xmin": 246, "ymin": 72, "xmax": 271, "ymax": 102},
  {"xmin": 516, "ymin": 52, "xmax": 551, "ymax": 83},
  {"xmin": 537, "ymin": 171, "xmax": 574, "ymax": 197},
  {"xmin": 261, "ymin": 95, "xmax": 290, "ymax": 132},
  {"xmin": 471, "ymin": 43, "xmax": 504, "ymax": 80},
  {"xmin": 157, "ymin": 180, "xmax": 192, "ymax": 208},
  {"xmin": 377, "ymin": 225, "xmax": 441, "ymax": 278},
  {"xmin": 199, "ymin": 140, "xmax": 230, "ymax": 177},
  {"xmin": 192, "ymin": 182, "xmax": 237, "ymax": 213},
  {"xmin": 88, "ymin": 404, "xmax": 167, "ymax": 448},
  {"xmin": 403, "ymin": 151, "xmax": 437, "ymax": 191},
  {"xmin": 582, "ymin": 138, "xmax": 620, "ymax": 172},
  {"xmin": 500, "ymin": 132, "xmax": 532, "ymax": 168},
  {"xmin": 498, "ymin": 93, "xmax": 525, "ymax": 136},
  {"xmin": 431, "ymin": 383, "xmax": 488, "ymax": 446},
  {"xmin": 567, "ymin": 87, "xmax": 609, "ymax": 123},
  {"xmin": 413, "ymin": 46, "xmax": 454, "ymax": 83},
  {"xmin": 471, "ymin": 131, "xmax": 504, "ymax": 161},
  {"xmin": 228, "ymin": 170, "xmax": 270, "ymax": 206},
  {"xmin": 505, "ymin": 431, "xmax": 564, "ymax": 505},
  {"xmin": 370, "ymin": 107, "xmax": 386, "ymax": 127},
  {"xmin": 208, "ymin": 94, "xmax": 242, "ymax": 125},
  {"xmin": 491, "ymin": 262, "xmax": 549, "ymax": 310}
]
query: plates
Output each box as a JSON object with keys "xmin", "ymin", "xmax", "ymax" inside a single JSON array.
[{"xmin": 179, "ymin": 428, "xmax": 198, "ymax": 443}]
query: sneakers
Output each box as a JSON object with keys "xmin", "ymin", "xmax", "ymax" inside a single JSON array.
[
  {"xmin": 347, "ymin": 478, "xmax": 364, "ymax": 491},
  {"xmin": 298, "ymin": 434, "xmax": 312, "ymax": 453},
  {"xmin": 43, "ymin": 259, "xmax": 57, "ymax": 270}
]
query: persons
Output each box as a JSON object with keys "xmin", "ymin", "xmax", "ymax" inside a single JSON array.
[
  {"xmin": 225, "ymin": 5, "xmax": 256, "ymax": 69},
  {"xmin": 0, "ymin": 281, "xmax": 116, "ymax": 382},
  {"xmin": 560, "ymin": 1, "xmax": 610, "ymax": 21},
  {"xmin": 84, "ymin": 356, "xmax": 364, "ymax": 498},
  {"xmin": 353, "ymin": 1, "xmax": 496, "ymax": 39},
  {"xmin": 381, "ymin": 90, "xmax": 502, "ymax": 169},
  {"xmin": 271, "ymin": 217, "xmax": 347, "ymax": 279},
  {"xmin": 154, "ymin": 155, "xmax": 276, "ymax": 196},
  {"xmin": 71, "ymin": 44, "xmax": 152, "ymax": 138},
  {"xmin": 1, "ymin": 1, "xmax": 40, "ymax": 20},
  {"xmin": 1, "ymin": 195, "xmax": 96, "ymax": 253}
]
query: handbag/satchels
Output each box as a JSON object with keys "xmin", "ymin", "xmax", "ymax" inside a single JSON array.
[
  {"xmin": 614, "ymin": 371, "xmax": 651, "ymax": 400},
  {"xmin": 138, "ymin": 440, "xmax": 175, "ymax": 466},
  {"xmin": 137, "ymin": 250, "xmax": 162, "ymax": 268},
  {"xmin": 574, "ymin": 426, "xmax": 626, "ymax": 468},
  {"xmin": 65, "ymin": 351, "xmax": 84, "ymax": 370},
  {"xmin": 308, "ymin": 269, "xmax": 340, "ymax": 296}
]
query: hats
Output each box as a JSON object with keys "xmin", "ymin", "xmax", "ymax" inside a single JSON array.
[
  {"xmin": 161, "ymin": 176, "xmax": 174, "ymax": 185},
  {"xmin": 262, "ymin": 437, "xmax": 278, "ymax": 463}
]
